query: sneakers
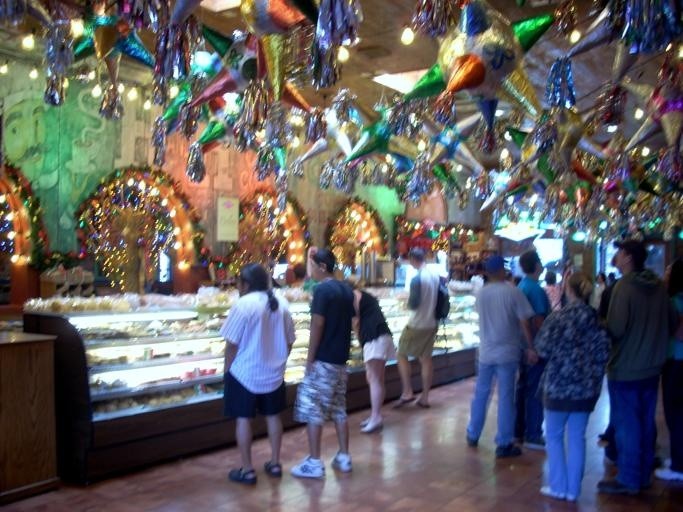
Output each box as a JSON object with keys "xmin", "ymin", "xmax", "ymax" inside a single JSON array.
[
  {"xmin": 596, "ymin": 481, "xmax": 643, "ymax": 498},
  {"xmin": 660, "ymin": 456, "xmax": 673, "ymax": 467},
  {"xmin": 331, "ymin": 451, "xmax": 353, "ymax": 473},
  {"xmin": 522, "ymin": 441, "xmax": 546, "ymax": 452},
  {"xmin": 511, "ymin": 437, "xmax": 523, "ymax": 445},
  {"xmin": 602, "ymin": 455, "xmax": 615, "ymax": 469},
  {"xmin": 540, "ymin": 485, "xmax": 565, "ymax": 500},
  {"xmin": 290, "ymin": 454, "xmax": 326, "ymax": 479},
  {"xmin": 653, "ymin": 467, "xmax": 682, "ymax": 483},
  {"xmin": 495, "ymin": 447, "xmax": 522, "ymax": 460},
  {"xmin": 466, "ymin": 435, "xmax": 477, "ymax": 447},
  {"xmin": 566, "ymin": 495, "xmax": 578, "ymax": 502}
]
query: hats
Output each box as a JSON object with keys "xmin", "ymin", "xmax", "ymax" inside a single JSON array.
[
  {"xmin": 481, "ymin": 253, "xmax": 510, "ymax": 274},
  {"xmin": 613, "ymin": 239, "xmax": 649, "ymax": 264}
]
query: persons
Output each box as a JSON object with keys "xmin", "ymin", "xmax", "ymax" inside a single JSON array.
[
  {"xmin": 515, "ymin": 250, "xmax": 553, "ymax": 451},
  {"xmin": 392, "ymin": 246, "xmax": 439, "ymax": 410},
  {"xmin": 221, "ymin": 262, "xmax": 297, "ymax": 485},
  {"xmin": 608, "ymin": 272, "xmax": 616, "ymax": 283},
  {"xmin": 344, "ymin": 279, "xmax": 395, "ymax": 433},
  {"xmin": 598, "ymin": 280, "xmax": 620, "ymax": 318},
  {"xmin": 541, "ymin": 271, "xmax": 565, "ymax": 312},
  {"xmin": 655, "ymin": 257, "xmax": 683, "ymax": 482},
  {"xmin": 597, "ymin": 239, "xmax": 669, "ymax": 496},
  {"xmin": 292, "ymin": 249, "xmax": 358, "ymax": 479},
  {"xmin": 597, "ymin": 252, "xmax": 628, "ymax": 464},
  {"xmin": 535, "ymin": 270, "xmax": 612, "ymax": 503},
  {"xmin": 588, "ymin": 272, "xmax": 606, "ymax": 311},
  {"xmin": 466, "ymin": 254, "xmax": 537, "ymax": 459}
]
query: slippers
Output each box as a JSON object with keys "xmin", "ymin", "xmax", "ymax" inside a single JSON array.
[
  {"xmin": 391, "ymin": 396, "xmax": 415, "ymax": 409},
  {"xmin": 416, "ymin": 399, "xmax": 431, "ymax": 408}
]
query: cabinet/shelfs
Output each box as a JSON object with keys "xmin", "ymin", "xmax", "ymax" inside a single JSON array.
[
  {"xmin": 0, "ymin": 326, "xmax": 63, "ymax": 505},
  {"xmin": 23, "ymin": 285, "xmax": 477, "ymax": 487}
]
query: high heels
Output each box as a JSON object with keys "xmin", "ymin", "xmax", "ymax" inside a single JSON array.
[
  {"xmin": 360, "ymin": 418, "xmax": 383, "ymax": 433},
  {"xmin": 359, "ymin": 417, "xmax": 369, "ymax": 427}
]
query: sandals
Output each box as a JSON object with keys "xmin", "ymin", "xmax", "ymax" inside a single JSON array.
[
  {"xmin": 264, "ymin": 462, "xmax": 282, "ymax": 478},
  {"xmin": 228, "ymin": 466, "xmax": 257, "ymax": 484}
]
